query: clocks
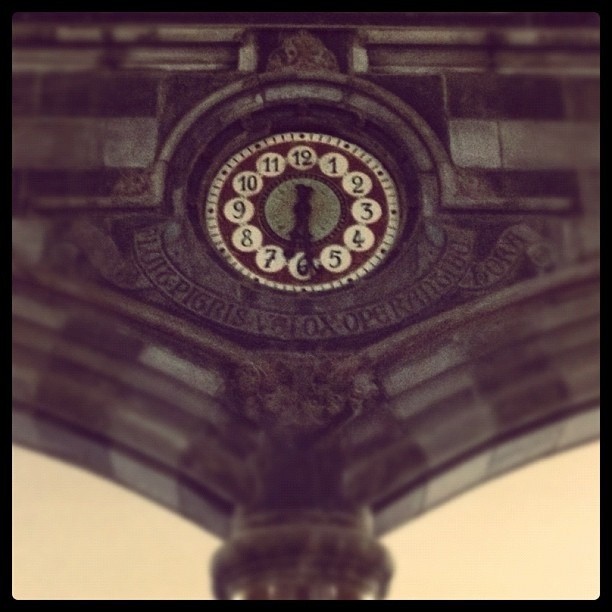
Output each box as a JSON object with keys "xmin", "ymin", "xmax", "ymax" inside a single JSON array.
[{"xmin": 204, "ymin": 130, "xmax": 401, "ymax": 295}]
